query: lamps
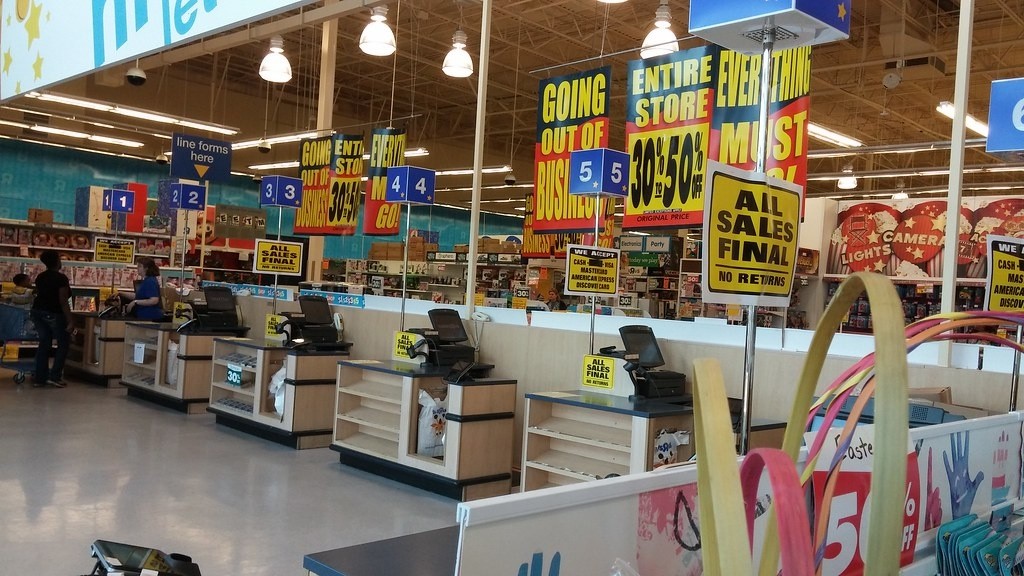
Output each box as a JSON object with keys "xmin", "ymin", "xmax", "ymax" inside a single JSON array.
[
  {"xmin": 936, "ymin": 101, "xmax": 988, "ymax": 138},
  {"xmin": 807, "ymin": 120, "xmax": 907, "ymax": 199},
  {"xmin": 442, "ymin": 23, "xmax": 473, "ymax": 77},
  {"xmin": 358, "ymin": 4, "xmax": 396, "ymax": 56},
  {"xmin": 24, "ymin": 90, "xmax": 536, "ymax": 211},
  {"xmin": 639, "ymin": 0, "xmax": 679, "ymax": 59},
  {"xmin": 259, "ymin": 34, "xmax": 292, "ymax": 83}
]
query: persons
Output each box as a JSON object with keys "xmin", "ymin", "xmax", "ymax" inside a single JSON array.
[
  {"xmin": 12, "ymin": 274, "xmax": 38, "ymax": 304},
  {"xmin": 30, "ymin": 251, "xmax": 73, "ymax": 387},
  {"xmin": 546, "ymin": 288, "xmax": 560, "ymax": 310},
  {"xmin": 110, "ymin": 258, "xmax": 162, "ymax": 320}
]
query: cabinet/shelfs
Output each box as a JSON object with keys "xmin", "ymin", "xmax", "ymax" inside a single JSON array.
[
  {"xmin": 196, "ymin": 244, "xmax": 254, "ymax": 273},
  {"xmin": 325, "ymin": 259, "xmax": 527, "ymax": 305},
  {"xmin": 205, "ymin": 334, "xmax": 353, "ymax": 449},
  {"xmin": 0, "ymin": 217, "xmax": 195, "ymax": 389},
  {"xmin": 326, "ymin": 358, "xmax": 518, "ymax": 502},
  {"xmin": 119, "ymin": 322, "xmax": 235, "ymax": 414},
  {"xmin": 616, "ymin": 259, "xmax": 818, "ymax": 330}
]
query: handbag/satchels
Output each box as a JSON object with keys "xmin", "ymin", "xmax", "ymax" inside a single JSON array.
[{"xmin": 31, "ymin": 295, "xmax": 63, "ymax": 329}]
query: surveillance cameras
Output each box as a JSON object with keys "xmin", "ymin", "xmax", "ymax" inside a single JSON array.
[
  {"xmin": 126, "ymin": 68, "xmax": 147, "ymax": 86},
  {"xmin": 258, "ymin": 142, "xmax": 271, "ymax": 153},
  {"xmin": 156, "ymin": 155, "xmax": 169, "ymax": 165},
  {"xmin": 505, "ymin": 176, "xmax": 516, "ymax": 185}
]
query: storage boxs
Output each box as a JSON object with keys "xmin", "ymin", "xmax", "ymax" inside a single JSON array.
[
  {"xmin": 682, "ymin": 282, "xmax": 694, "ymax": 297},
  {"xmin": 349, "ymin": 236, "xmax": 524, "ymax": 308},
  {"xmin": 27, "ymin": 208, "xmax": 53, "ymax": 223}
]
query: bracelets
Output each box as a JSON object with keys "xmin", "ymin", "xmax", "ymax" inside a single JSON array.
[{"xmin": 135, "ymin": 300, "xmax": 137, "ymax": 304}]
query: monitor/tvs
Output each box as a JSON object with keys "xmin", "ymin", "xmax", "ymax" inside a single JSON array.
[
  {"xmin": 618, "ymin": 325, "xmax": 665, "ymax": 369},
  {"xmin": 204, "ymin": 286, "xmax": 234, "ymax": 312},
  {"xmin": 133, "ymin": 280, "xmax": 145, "ymax": 291},
  {"xmin": 298, "ymin": 295, "xmax": 333, "ymax": 327},
  {"xmin": 428, "ymin": 309, "xmax": 468, "ymax": 345}
]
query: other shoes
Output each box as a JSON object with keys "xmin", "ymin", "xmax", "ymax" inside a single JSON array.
[
  {"xmin": 33, "ymin": 378, "xmax": 46, "ymax": 386},
  {"xmin": 46, "ymin": 375, "xmax": 67, "ymax": 388}
]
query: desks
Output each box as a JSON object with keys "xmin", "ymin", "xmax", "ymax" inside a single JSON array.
[{"xmin": 518, "ymin": 391, "xmax": 695, "ymax": 492}]
